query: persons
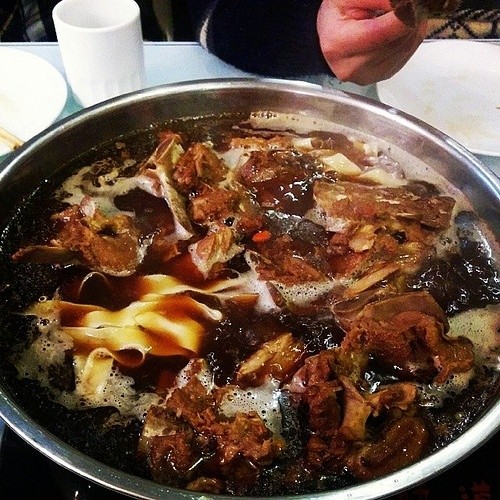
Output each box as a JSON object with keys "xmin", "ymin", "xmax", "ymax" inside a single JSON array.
[{"xmin": 197, "ymin": 0, "xmax": 428, "ymax": 88}]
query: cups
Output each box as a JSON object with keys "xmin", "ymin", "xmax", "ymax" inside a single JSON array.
[{"xmin": 51, "ymin": 0, "xmax": 147, "ymax": 109}]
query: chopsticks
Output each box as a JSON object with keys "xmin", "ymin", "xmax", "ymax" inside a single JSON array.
[{"xmin": 0, "ymin": 126, "xmax": 24, "ymax": 150}]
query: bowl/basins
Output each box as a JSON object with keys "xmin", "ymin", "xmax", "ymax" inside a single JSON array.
[{"xmin": 0, "ymin": 77, "xmax": 500, "ymax": 500}]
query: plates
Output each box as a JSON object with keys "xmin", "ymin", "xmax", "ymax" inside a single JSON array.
[{"xmin": 0, "ymin": 47, "xmax": 68, "ymax": 159}]
